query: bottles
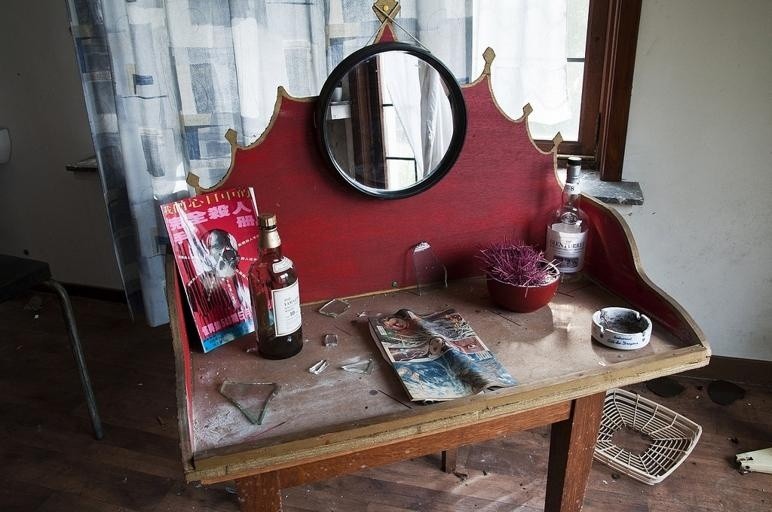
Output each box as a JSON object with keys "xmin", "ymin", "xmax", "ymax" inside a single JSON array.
[
  {"xmin": 250, "ymin": 214, "xmax": 307, "ymax": 358},
  {"xmin": 541, "ymin": 152, "xmax": 590, "ymax": 284}
]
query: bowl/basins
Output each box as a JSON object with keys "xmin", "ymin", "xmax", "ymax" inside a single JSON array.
[{"xmin": 486, "ymin": 259, "xmax": 560, "ymax": 313}]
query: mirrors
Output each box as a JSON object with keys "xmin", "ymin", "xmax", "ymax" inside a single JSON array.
[{"xmin": 312, "ymin": 38, "xmax": 467, "ymax": 201}]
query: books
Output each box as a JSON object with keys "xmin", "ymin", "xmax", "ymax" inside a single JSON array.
[
  {"xmin": 158, "ymin": 186, "xmax": 268, "ymax": 353},
  {"xmin": 366, "ymin": 306, "xmax": 516, "ymax": 403}
]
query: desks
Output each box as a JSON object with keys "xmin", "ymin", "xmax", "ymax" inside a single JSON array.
[{"xmin": 173, "ymin": 192, "xmax": 711, "ymax": 511}]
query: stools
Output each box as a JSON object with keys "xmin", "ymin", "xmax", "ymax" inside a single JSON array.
[{"xmin": 0, "ymin": 250, "xmax": 107, "ymax": 444}]
